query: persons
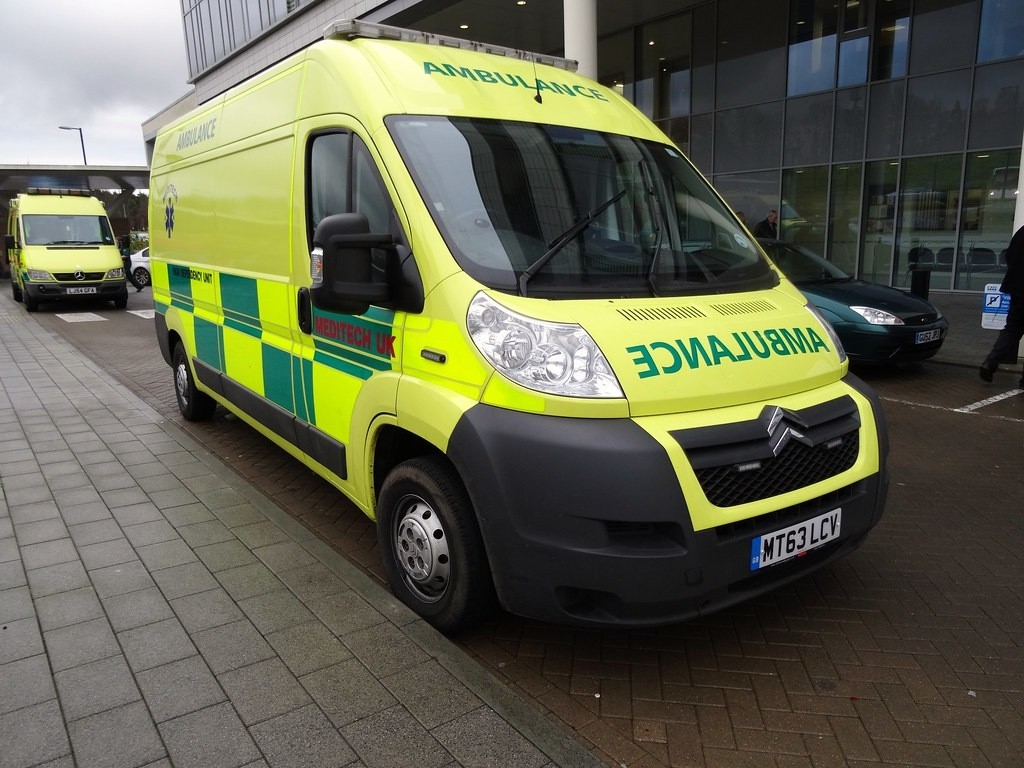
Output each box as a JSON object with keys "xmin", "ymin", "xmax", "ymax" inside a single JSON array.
[
  {"xmin": 735, "ymin": 211, "xmax": 744, "ymax": 223},
  {"xmin": 752, "ymin": 210, "xmax": 777, "ymax": 240},
  {"xmin": 979, "ymin": 225, "xmax": 1024, "ymax": 390},
  {"xmin": 907, "ymin": 234, "xmax": 936, "ymax": 299},
  {"xmin": 118, "ymin": 233, "xmax": 144, "ymax": 292}
]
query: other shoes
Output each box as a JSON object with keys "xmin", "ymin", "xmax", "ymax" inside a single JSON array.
[
  {"xmin": 980, "ymin": 361, "xmax": 997, "ymax": 382},
  {"xmin": 137, "ymin": 285, "xmax": 145, "ymax": 292},
  {"xmin": 1019, "ymin": 378, "xmax": 1024, "ymax": 390}
]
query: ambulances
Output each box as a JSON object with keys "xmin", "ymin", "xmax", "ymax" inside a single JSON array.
[
  {"xmin": 147, "ymin": 36, "xmax": 890, "ymax": 633},
  {"xmin": 5, "ymin": 185, "xmax": 129, "ymax": 313}
]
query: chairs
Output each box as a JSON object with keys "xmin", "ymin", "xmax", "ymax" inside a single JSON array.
[
  {"xmin": 966, "ymin": 248, "xmax": 1000, "ymax": 292},
  {"xmin": 904, "ymin": 247, "xmax": 936, "ymax": 289},
  {"xmin": 997, "ymin": 248, "xmax": 1009, "ymax": 290},
  {"xmin": 934, "ymin": 248, "xmax": 968, "ymax": 290}
]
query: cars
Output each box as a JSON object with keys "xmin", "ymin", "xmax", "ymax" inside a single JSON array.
[
  {"xmin": 750, "ymin": 236, "xmax": 950, "ymax": 375},
  {"xmin": 124, "ymin": 245, "xmax": 156, "ymax": 285}
]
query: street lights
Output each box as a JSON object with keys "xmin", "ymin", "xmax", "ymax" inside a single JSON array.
[{"xmin": 59, "ymin": 123, "xmax": 88, "ymax": 165}]
